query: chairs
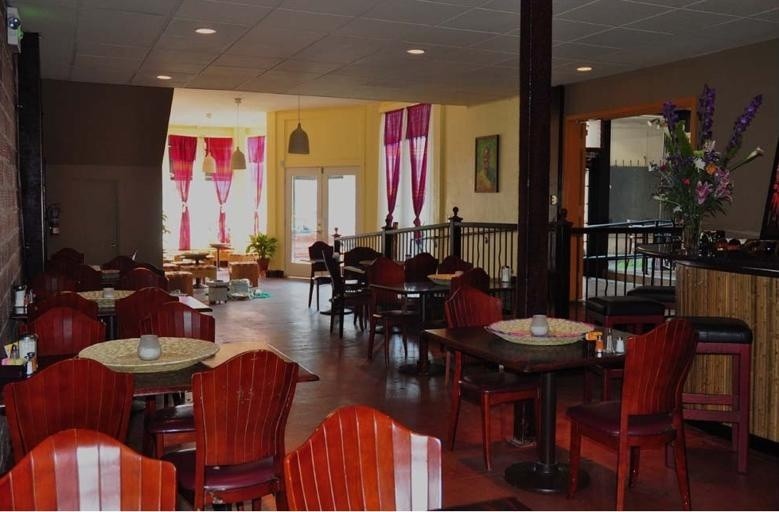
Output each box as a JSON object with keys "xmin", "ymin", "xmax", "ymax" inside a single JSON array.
[
  {"xmin": 447, "ymin": 286, "xmax": 544, "ymax": 472},
  {"xmin": 0, "ymin": 430, "xmax": 176, "ymax": 511},
  {"xmin": 323, "ymin": 248, "xmax": 369, "ymax": 335},
  {"xmin": 309, "ymin": 242, "xmax": 334, "ymax": 309},
  {"xmin": 26, "ymin": 271, "xmax": 76, "ymax": 292},
  {"xmin": 368, "ymin": 258, "xmax": 425, "ymax": 365},
  {"xmin": 26, "ymin": 293, "xmax": 100, "ymax": 329},
  {"xmin": 452, "ymin": 268, "xmax": 492, "ymax": 294},
  {"xmin": 283, "ymin": 407, "xmax": 441, "ymax": 512},
  {"xmin": 70, "ymin": 266, "xmax": 103, "ymax": 290},
  {"xmin": 567, "ymin": 318, "xmax": 699, "ymax": 512},
  {"xmin": 162, "ymin": 351, "xmax": 298, "ymax": 511},
  {"xmin": 138, "ymin": 304, "xmax": 215, "ymax": 344},
  {"xmin": 115, "ymin": 288, "xmax": 178, "ymax": 339},
  {"xmin": 17, "ymin": 307, "xmax": 106, "ymax": 356},
  {"xmin": 6, "ymin": 358, "xmax": 135, "ymax": 468},
  {"xmin": 120, "ymin": 268, "xmax": 166, "ymax": 291},
  {"xmin": 343, "ymin": 247, "xmax": 379, "ymax": 282},
  {"xmin": 407, "ymin": 253, "xmax": 437, "ymax": 278},
  {"xmin": 53, "ymin": 249, "xmax": 81, "ymax": 268}
]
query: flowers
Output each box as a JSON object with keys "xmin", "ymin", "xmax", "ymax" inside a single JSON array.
[{"xmin": 650, "ymin": 88, "xmax": 763, "ymax": 214}]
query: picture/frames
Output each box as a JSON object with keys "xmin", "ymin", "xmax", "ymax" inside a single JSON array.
[{"xmin": 472, "ymin": 136, "xmax": 501, "ymax": 194}]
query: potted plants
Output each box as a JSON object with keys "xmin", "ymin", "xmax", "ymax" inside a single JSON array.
[{"xmin": 248, "ymin": 235, "xmax": 278, "ymax": 273}]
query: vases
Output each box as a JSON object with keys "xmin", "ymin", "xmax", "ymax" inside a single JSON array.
[{"xmin": 679, "ymin": 213, "xmax": 699, "ymax": 256}]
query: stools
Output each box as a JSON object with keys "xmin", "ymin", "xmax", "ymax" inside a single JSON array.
[
  {"xmin": 680, "ymin": 316, "xmax": 753, "ymax": 475},
  {"xmin": 587, "ymin": 296, "xmax": 665, "ymax": 400}
]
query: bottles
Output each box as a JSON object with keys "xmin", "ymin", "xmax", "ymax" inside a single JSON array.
[
  {"xmin": 606, "ymin": 328, "xmax": 615, "ymax": 353},
  {"xmin": 10, "ymin": 345, "xmax": 18, "ymax": 360},
  {"xmin": 26, "ymin": 352, "xmax": 36, "ymax": 372},
  {"xmin": 594, "ymin": 335, "xmax": 604, "ymax": 353},
  {"xmin": 616, "ymin": 336, "xmax": 624, "ymax": 353},
  {"xmin": 25, "ymin": 356, "xmax": 33, "ymax": 375},
  {"xmin": 500, "ymin": 265, "xmax": 510, "ymax": 282}
]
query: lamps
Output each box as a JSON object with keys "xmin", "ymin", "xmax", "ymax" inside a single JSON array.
[
  {"xmin": 290, "ymin": 96, "xmax": 311, "ymax": 153},
  {"xmin": 202, "ymin": 114, "xmax": 217, "ymax": 174},
  {"xmin": 230, "ymin": 98, "xmax": 246, "ymax": 170}
]
